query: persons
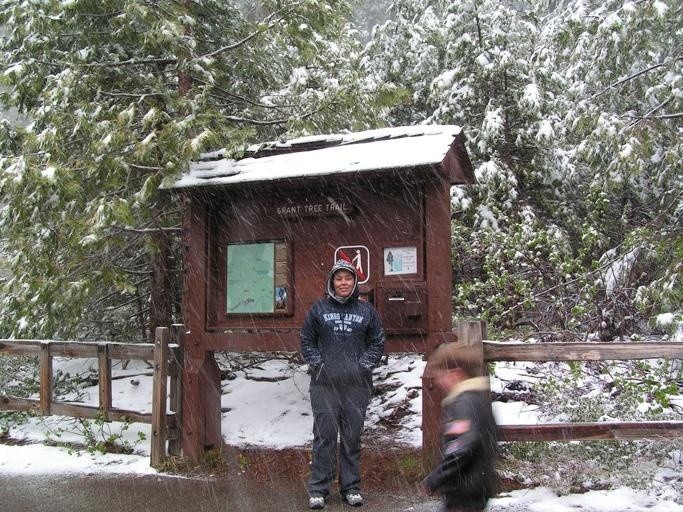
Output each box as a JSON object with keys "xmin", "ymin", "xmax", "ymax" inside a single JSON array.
[
  {"xmin": 301, "ymin": 259, "xmax": 386, "ymax": 509},
  {"xmin": 418, "ymin": 341, "xmax": 499, "ymax": 512}
]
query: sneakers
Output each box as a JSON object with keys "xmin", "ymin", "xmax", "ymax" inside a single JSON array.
[
  {"xmin": 309, "ymin": 494, "xmax": 324, "ymax": 509},
  {"xmin": 345, "ymin": 491, "xmax": 363, "ymax": 506}
]
